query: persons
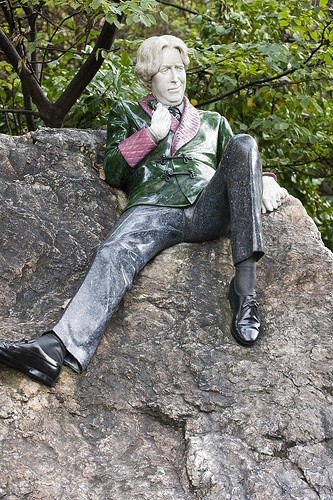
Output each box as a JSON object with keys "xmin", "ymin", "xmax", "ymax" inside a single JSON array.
[{"xmin": 0, "ymin": 35, "xmax": 289, "ymax": 390}]
